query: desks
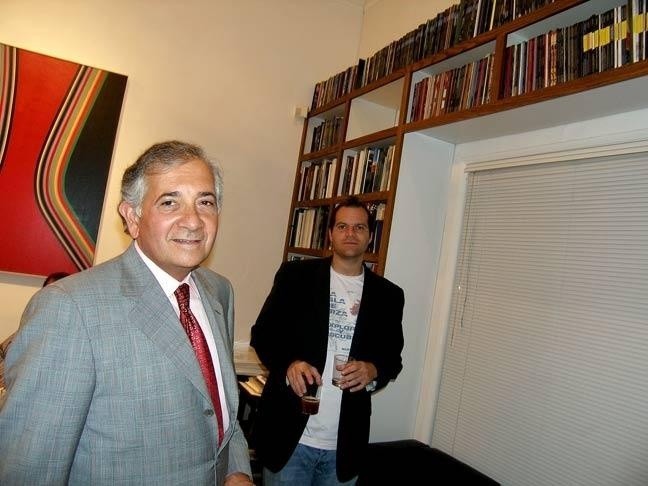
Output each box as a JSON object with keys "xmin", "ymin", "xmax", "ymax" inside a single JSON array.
[{"xmin": 237, "ymin": 382, "xmax": 263, "ymax": 448}]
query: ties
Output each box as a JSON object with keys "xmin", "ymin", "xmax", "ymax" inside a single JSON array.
[{"xmin": 174, "ymin": 283, "xmax": 224, "ymax": 450}]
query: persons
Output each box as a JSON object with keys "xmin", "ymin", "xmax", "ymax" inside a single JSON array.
[
  {"xmin": 0, "ymin": 139, "xmax": 255, "ymax": 486},
  {"xmin": 0, "ymin": 272, "xmax": 74, "ymax": 360},
  {"xmin": 248, "ymin": 197, "xmax": 405, "ymax": 486}
]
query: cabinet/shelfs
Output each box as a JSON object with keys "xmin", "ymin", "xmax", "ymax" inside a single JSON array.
[
  {"xmin": 399, "ymin": 0, "xmax": 647, "ymax": 134},
  {"xmin": 278, "ymin": 66, "xmax": 412, "ymax": 279}
]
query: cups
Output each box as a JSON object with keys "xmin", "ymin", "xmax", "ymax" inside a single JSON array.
[
  {"xmin": 300, "ymin": 373, "xmax": 323, "ymax": 415},
  {"xmin": 331, "ymin": 353, "xmax": 358, "ymax": 387}
]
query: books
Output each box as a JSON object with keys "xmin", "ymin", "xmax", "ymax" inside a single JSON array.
[
  {"xmin": 289, "ymin": 201, "xmax": 386, "ymax": 254},
  {"xmin": 409, "ymin": 0, "xmax": 646, "ymax": 122},
  {"xmin": 296, "ymin": 144, "xmax": 395, "ymax": 201},
  {"xmin": 308, "ymin": 34, "xmax": 412, "ymax": 110},
  {"xmin": 233, "ymin": 341, "xmax": 271, "ymax": 396},
  {"xmin": 311, "ymin": 116, "xmax": 343, "ymax": 153}
]
